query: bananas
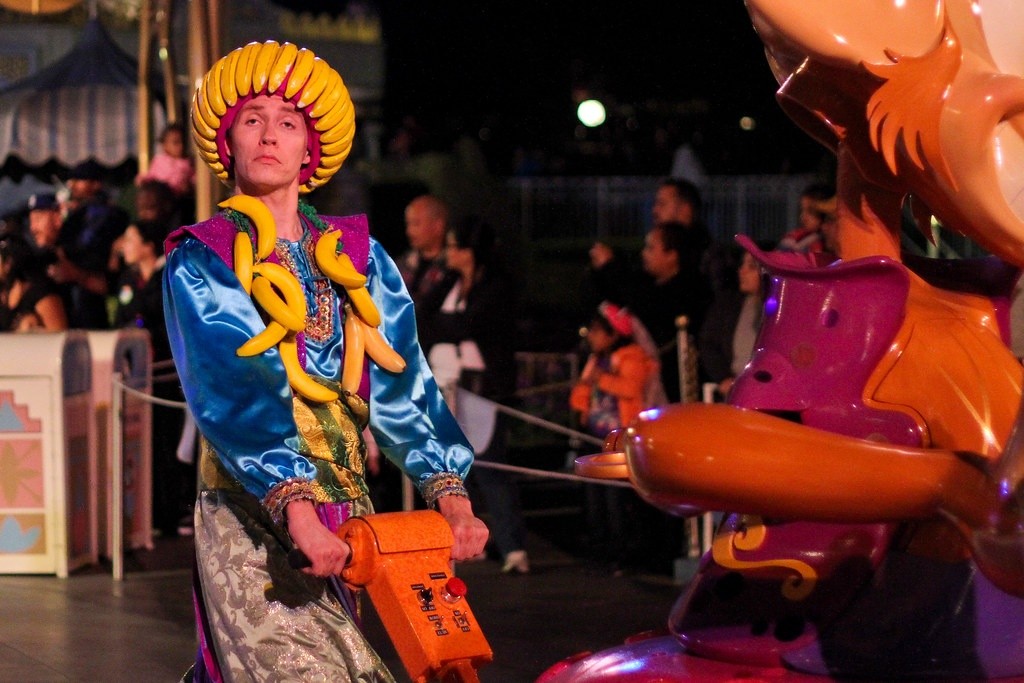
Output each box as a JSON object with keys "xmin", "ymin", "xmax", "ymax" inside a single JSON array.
[
  {"xmin": 190, "ymin": 40, "xmax": 356, "ymax": 193},
  {"xmin": 216, "ymin": 195, "xmax": 406, "ymax": 403}
]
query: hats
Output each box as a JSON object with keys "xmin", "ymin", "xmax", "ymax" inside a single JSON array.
[{"xmin": 190, "ymin": 40, "xmax": 355, "ymax": 194}]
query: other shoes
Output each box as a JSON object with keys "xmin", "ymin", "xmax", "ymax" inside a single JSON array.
[{"xmin": 502, "ymin": 550, "xmax": 528, "ymax": 575}]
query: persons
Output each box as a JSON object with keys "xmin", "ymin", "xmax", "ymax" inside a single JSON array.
[
  {"xmin": 571, "ymin": 179, "xmax": 837, "ymax": 576},
  {"xmin": 0, "ymin": 126, "xmax": 204, "ymax": 538},
  {"xmin": 162, "ymin": 40, "xmax": 489, "ymax": 683},
  {"xmin": 391, "ymin": 197, "xmax": 534, "ymax": 576}
]
